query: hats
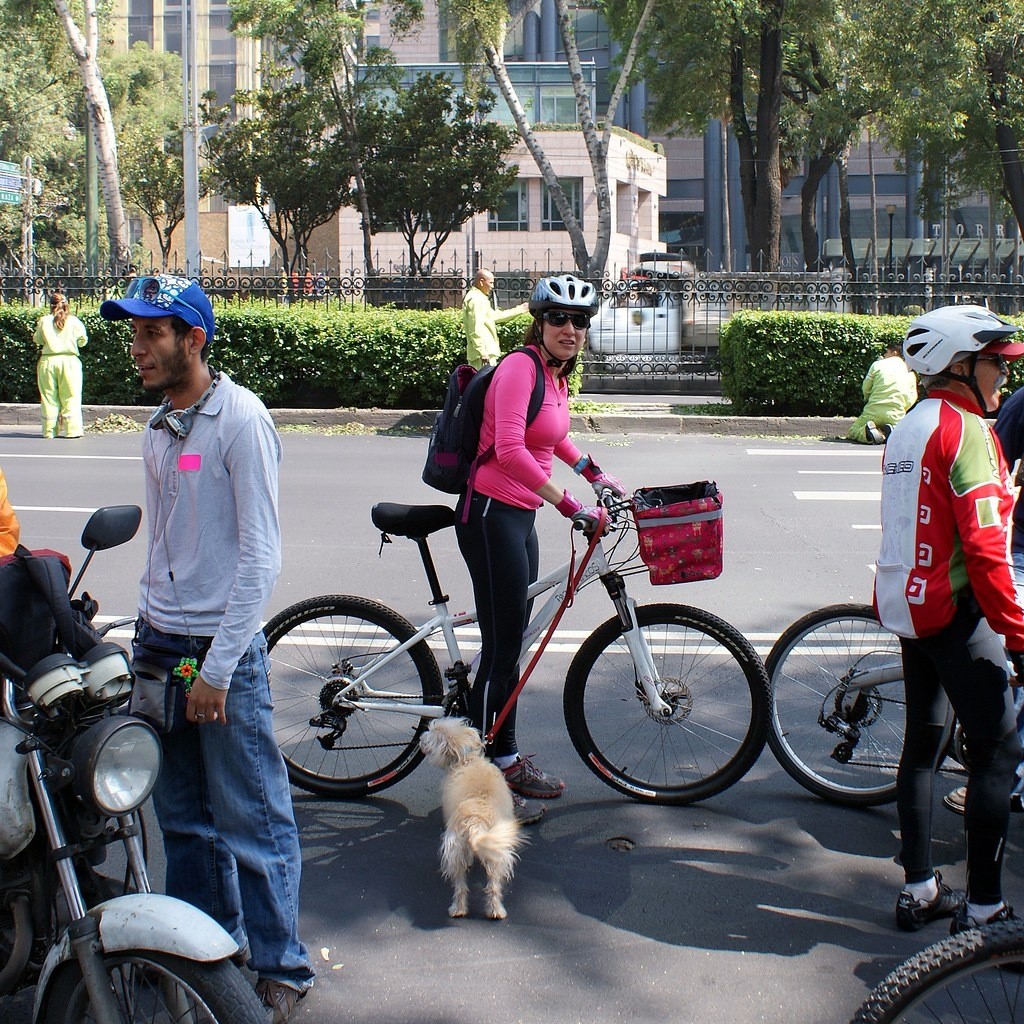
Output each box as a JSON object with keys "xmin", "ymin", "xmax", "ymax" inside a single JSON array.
[
  {"xmin": 978, "ymin": 341, "xmax": 1024, "ymax": 362},
  {"xmin": 100, "ymin": 275, "xmax": 215, "ymax": 344}
]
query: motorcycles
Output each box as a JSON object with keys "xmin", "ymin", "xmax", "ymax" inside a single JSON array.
[{"xmin": 0, "ymin": 502, "xmax": 270, "ymax": 1023}]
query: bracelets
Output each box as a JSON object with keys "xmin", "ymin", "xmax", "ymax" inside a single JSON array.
[{"xmin": 573, "ymin": 454, "xmax": 589, "ymax": 476}]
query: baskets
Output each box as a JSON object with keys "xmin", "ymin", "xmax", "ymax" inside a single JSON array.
[{"xmin": 631, "ymin": 481, "xmax": 724, "ymax": 584}]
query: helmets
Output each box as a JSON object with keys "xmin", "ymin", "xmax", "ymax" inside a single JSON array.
[
  {"xmin": 903, "ymin": 304, "xmax": 1021, "ymax": 375},
  {"xmin": 529, "ymin": 275, "xmax": 598, "ymax": 316}
]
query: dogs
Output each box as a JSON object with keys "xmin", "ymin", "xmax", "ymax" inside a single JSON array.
[{"xmin": 419, "ymin": 717, "xmax": 531, "ymax": 919}]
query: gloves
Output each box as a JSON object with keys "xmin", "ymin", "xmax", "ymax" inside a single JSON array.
[
  {"xmin": 590, "ymin": 474, "xmax": 627, "ymax": 500},
  {"xmin": 571, "ymin": 504, "xmax": 612, "ymax": 532}
]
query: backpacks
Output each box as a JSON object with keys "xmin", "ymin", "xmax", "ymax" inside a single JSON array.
[{"xmin": 422, "ymin": 347, "xmax": 545, "ymax": 495}]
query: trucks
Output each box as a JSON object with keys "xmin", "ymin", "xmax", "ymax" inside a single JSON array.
[{"xmin": 587, "ymin": 257, "xmax": 851, "ymax": 374}]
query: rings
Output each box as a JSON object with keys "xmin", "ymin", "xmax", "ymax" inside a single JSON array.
[{"xmin": 197, "ymin": 713, "xmax": 205, "ymax": 717}]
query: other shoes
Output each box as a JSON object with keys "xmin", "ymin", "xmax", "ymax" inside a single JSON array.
[
  {"xmin": 883, "ymin": 424, "xmax": 892, "ymax": 444},
  {"xmin": 866, "ymin": 421, "xmax": 882, "ymax": 444}
]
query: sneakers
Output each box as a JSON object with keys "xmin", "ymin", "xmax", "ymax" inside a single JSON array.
[
  {"xmin": 254, "ymin": 976, "xmax": 307, "ymax": 1024},
  {"xmin": 508, "ymin": 789, "xmax": 546, "ymax": 826},
  {"xmin": 503, "ymin": 753, "xmax": 564, "ymax": 796},
  {"xmin": 897, "ymin": 869, "xmax": 966, "ymax": 931},
  {"xmin": 950, "ymin": 902, "xmax": 1024, "ymax": 973},
  {"xmin": 229, "ymin": 948, "xmax": 247, "ymax": 968},
  {"xmin": 943, "ymin": 787, "xmax": 966, "ymax": 814}
]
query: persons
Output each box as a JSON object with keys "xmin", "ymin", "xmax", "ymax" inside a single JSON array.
[
  {"xmin": 455, "ymin": 275, "xmax": 626, "ymax": 825},
  {"xmin": 0, "ymin": 465, "xmax": 20, "ymax": 558},
  {"xmin": 100, "ymin": 273, "xmax": 317, "ymax": 1024},
  {"xmin": 943, "ymin": 386, "xmax": 1024, "ymax": 814},
  {"xmin": 33, "ymin": 293, "xmax": 89, "ymax": 439},
  {"xmin": 873, "ymin": 307, "xmax": 1024, "ymax": 975},
  {"xmin": 463, "ymin": 270, "xmax": 529, "ymax": 372},
  {"xmin": 849, "ymin": 342, "xmax": 919, "ymax": 445}
]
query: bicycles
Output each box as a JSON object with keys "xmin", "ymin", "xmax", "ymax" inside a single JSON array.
[
  {"xmin": 260, "ymin": 478, "xmax": 774, "ymax": 807},
  {"xmin": 762, "ymin": 601, "xmax": 975, "ymax": 808},
  {"xmin": 846, "ymin": 918, "xmax": 1024, "ymax": 1023}
]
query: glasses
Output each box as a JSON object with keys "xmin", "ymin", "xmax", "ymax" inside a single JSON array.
[
  {"xmin": 124, "ymin": 277, "xmax": 207, "ymax": 331},
  {"xmin": 975, "ymin": 354, "xmax": 1005, "ymax": 371},
  {"xmin": 536, "ymin": 311, "xmax": 591, "ymax": 329}
]
